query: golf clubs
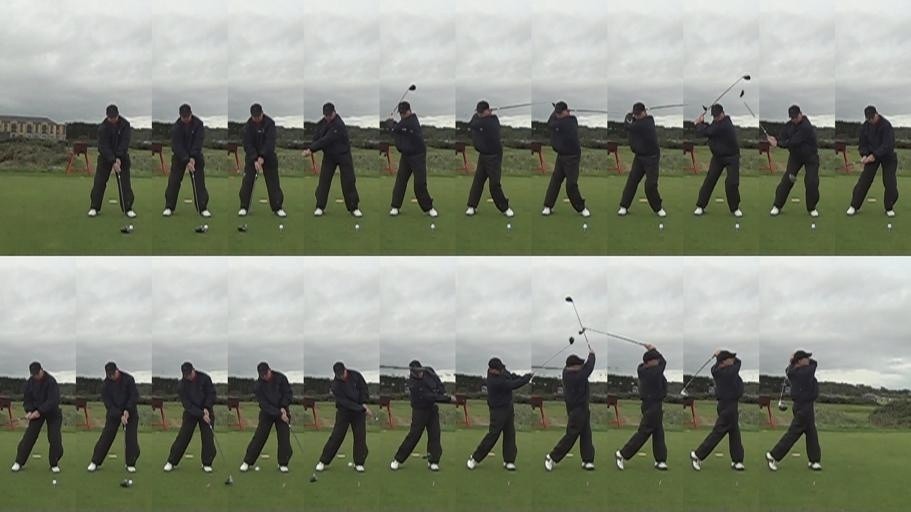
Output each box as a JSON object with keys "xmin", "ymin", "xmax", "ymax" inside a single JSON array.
[
  {"xmin": 121, "ymin": 296, "xmax": 787, "ymax": 492},
  {"xmin": 117, "ymin": 83, "xmax": 429, "ymax": 235},
  {"xmin": 702, "ymin": 74, "xmax": 771, "ymax": 139}
]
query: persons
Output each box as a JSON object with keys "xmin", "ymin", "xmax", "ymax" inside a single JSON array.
[
  {"xmin": 384, "ymin": 101, "xmax": 438, "ymax": 218},
  {"xmin": 541, "ymin": 101, "xmax": 591, "ymax": 217},
  {"xmin": 617, "ymin": 102, "xmax": 666, "ymax": 218},
  {"xmin": 301, "ymin": 103, "xmax": 363, "ymax": 217},
  {"xmin": 846, "ymin": 105, "xmax": 899, "ymax": 218},
  {"xmin": 237, "ymin": 104, "xmax": 287, "ymax": 217},
  {"xmin": 766, "ymin": 104, "xmax": 821, "ymax": 218},
  {"xmin": 693, "ymin": 103, "xmax": 743, "ymax": 218},
  {"xmin": 465, "ymin": 100, "xmax": 514, "ymax": 218},
  {"xmin": 162, "ymin": 102, "xmax": 212, "ymax": 217},
  {"xmin": 11, "ymin": 347, "xmax": 826, "ymax": 474},
  {"xmin": 88, "ymin": 104, "xmax": 136, "ymax": 218}
]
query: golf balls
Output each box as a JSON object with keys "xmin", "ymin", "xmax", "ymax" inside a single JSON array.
[
  {"xmin": 126, "ymin": 224, "xmax": 894, "ymax": 229},
  {"xmin": 128, "ymin": 479, "xmax": 132, "ymax": 484},
  {"xmin": 53, "ymin": 480, "xmax": 56, "ymax": 484},
  {"xmin": 255, "ymin": 466, "xmax": 259, "ymax": 470}
]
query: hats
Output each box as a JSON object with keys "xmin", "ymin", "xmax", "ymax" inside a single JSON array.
[
  {"xmin": 474, "ymin": 101, "xmax": 489, "ymax": 113},
  {"xmin": 182, "ymin": 362, "xmax": 193, "ymax": 376},
  {"xmin": 106, "ymin": 105, "xmax": 119, "ymax": 117},
  {"xmin": 333, "ymin": 361, "xmax": 345, "ymax": 377},
  {"xmin": 633, "ymin": 103, "xmax": 645, "ymax": 115},
  {"xmin": 30, "ymin": 361, "xmax": 41, "ymax": 375},
  {"xmin": 566, "ymin": 355, "xmax": 585, "ymax": 367},
  {"xmin": 250, "ymin": 104, "xmax": 262, "ymax": 117},
  {"xmin": 488, "ymin": 358, "xmax": 505, "ymax": 369},
  {"xmin": 711, "ymin": 104, "xmax": 723, "ymax": 117},
  {"xmin": 717, "ymin": 351, "xmax": 736, "ymax": 361},
  {"xmin": 397, "ymin": 101, "xmax": 410, "ymax": 113},
  {"xmin": 323, "ymin": 103, "xmax": 334, "ymax": 115},
  {"xmin": 410, "ymin": 360, "xmax": 421, "ymax": 374},
  {"xmin": 257, "ymin": 362, "xmax": 268, "ymax": 376},
  {"xmin": 788, "ymin": 105, "xmax": 800, "ymax": 117},
  {"xmin": 105, "ymin": 362, "xmax": 117, "ymax": 376},
  {"xmin": 555, "ymin": 102, "xmax": 568, "ymax": 113},
  {"xmin": 865, "ymin": 106, "xmax": 876, "ymax": 119},
  {"xmin": 643, "ymin": 351, "xmax": 660, "ymax": 362},
  {"xmin": 794, "ymin": 350, "xmax": 812, "ymax": 361},
  {"xmin": 179, "ymin": 104, "xmax": 191, "ymax": 117}
]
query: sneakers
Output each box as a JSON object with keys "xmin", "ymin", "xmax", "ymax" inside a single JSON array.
[
  {"xmin": 544, "ymin": 453, "xmax": 554, "ymax": 471},
  {"xmin": 390, "ymin": 208, "xmax": 398, "ymax": 215},
  {"xmin": 581, "ymin": 207, "xmax": 591, "ymax": 217},
  {"xmin": 690, "ymin": 450, "xmax": 701, "ymax": 471},
  {"xmin": 238, "ymin": 209, "xmax": 247, "ymax": 215},
  {"xmin": 809, "ymin": 209, "xmax": 819, "ymax": 217},
  {"xmin": 240, "ymin": 461, "xmax": 249, "ymax": 472},
  {"xmin": 581, "ymin": 461, "xmax": 595, "ymax": 470},
  {"xmin": 466, "ymin": 207, "xmax": 478, "ymax": 216},
  {"xmin": 126, "ymin": 210, "xmax": 136, "ymax": 218},
  {"xmin": 51, "ymin": 465, "xmax": 61, "ymax": 473},
  {"xmin": 201, "ymin": 210, "xmax": 211, "ymax": 217},
  {"xmin": 542, "ymin": 207, "xmax": 553, "ymax": 215},
  {"xmin": 11, "ymin": 462, "xmax": 22, "ymax": 471},
  {"xmin": 427, "ymin": 461, "xmax": 439, "ymax": 470},
  {"xmin": 316, "ymin": 461, "xmax": 325, "ymax": 472},
  {"xmin": 467, "ymin": 454, "xmax": 477, "ymax": 470},
  {"xmin": 847, "ymin": 206, "xmax": 858, "ymax": 215},
  {"xmin": 275, "ymin": 209, "xmax": 287, "ymax": 217},
  {"xmin": 352, "ymin": 209, "xmax": 362, "ymax": 217},
  {"xmin": 766, "ymin": 451, "xmax": 777, "ymax": 471},
  {"xmin": 731, "ymin": 461, "xmax": 744, "ymax": 470},
  {"xmin": 808, "ymin": 461, "xmax": 822, "ymax": 470},
  {"xmin": 770, "ymin": 206, "xmax": 780, "ymax": 216},
  {"xmin": 885, "ymin": 210, "xmax": 895, "ymax": 217},
  {"xmin": 125, "ymin": 464, "xmax": 136, "ymax": 473},
  {"xmin": 354, "ymin": 464, "xmax": 365, "ymax": 472},
  {"xmin": 391, "ymin": 459, "xmax": 400, "ymax": 471},
  {"xmin": 617, "ymin": 206, "xmax": 628, "ymax": 215},
  {"xmin": 503, "ymin": 461, "xmax": 516, "ymax": 470},
  {"xmin": 313, "ymin": 208, "xmax": 323, "ymax": 216},
  {"xmin": 277, "ymin": 463, "xmax": 288, "ymax": 472},
  {"xmin": 87, "ymin": 208, "xmax": 97, "ymax": 216},
  {"xmin": 657, "ymin": 208, "xmax": 666, "ymax": 216},
  {"xmin": 693, "ymin": 207, "xmax": 704, "ymax": 215},
  {"xmin": 201, "ymin": 464, "xmax": 213, "ymax": 472},
  {"xmin": 654, "ymin": 460, "xmax": 669, "ymax": 470},
  {"xmin": 429, "ymin": 208, "xmax": 438, "ymax": 217},
  {"xmin": 162, "ymin": 208, "xmax": 172, "ymax": 216},
  {"xmin": 163, "ymin": 462, "xmax": 174, "ymax": 471},
  {"xmin": 504, "ymin": 207, "xmax": 513, "ymax": 217},
  {"xmin": 87, "ymin": 462, "xmax": 101, "ymax": 471},
  {"xmin": 615, "ymin": 449, "xmax": 624, "ymax": 471},
  {"xmin": 734, "ymin": 208, "xmax": 743, "ymax": 218}
]
